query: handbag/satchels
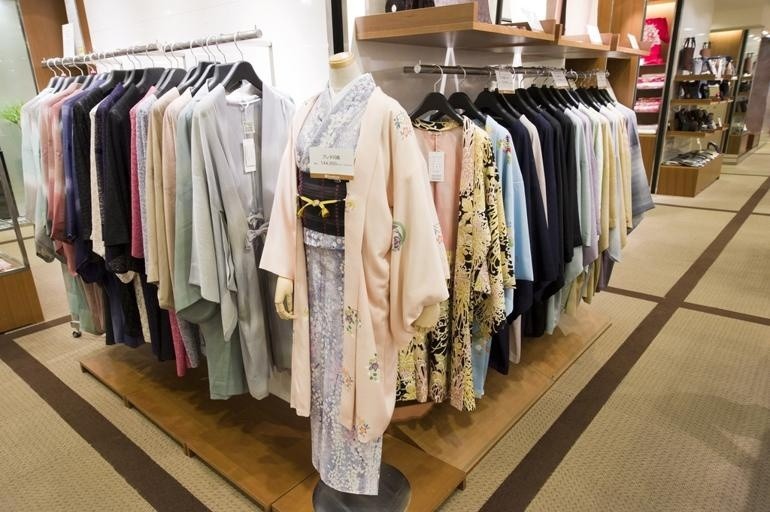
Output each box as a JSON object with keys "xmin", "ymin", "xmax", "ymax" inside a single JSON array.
[
  {"xmin": 678, "ymin": 47, "xmax": 695, "ymax": 70},
  {"xmin": 699, "ymin": 48, "xmax": 713, "ymax": 61},
  {"xmin": 743, "ymin": 57, "xmax": 753, "ymax": 75}
]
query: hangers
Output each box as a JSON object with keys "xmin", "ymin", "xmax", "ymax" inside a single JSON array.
[
  {"xmin": 47, "ymin": 30, "xmax": 262, "ymax": 95},
  {"xmin": 408, "ymin": 64, "xmax": 615, "ymax": 127}
]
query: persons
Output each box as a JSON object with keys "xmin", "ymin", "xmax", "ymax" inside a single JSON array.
[{"xmin": 258, "ymin": 52, "xmax": 450, "ymax": 497}]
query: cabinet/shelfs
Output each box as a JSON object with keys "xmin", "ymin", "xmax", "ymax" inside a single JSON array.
[
  {"xmin": 726, "ymin": 76, "xmax": 761, "ymax": 155},
  {"xmin": 657, "ymin": 76, "xmax": 733, "ymax": 198},
  {"xmin": 634, "ymin": 0, "xmax": 676, "ymax": 187}
]
